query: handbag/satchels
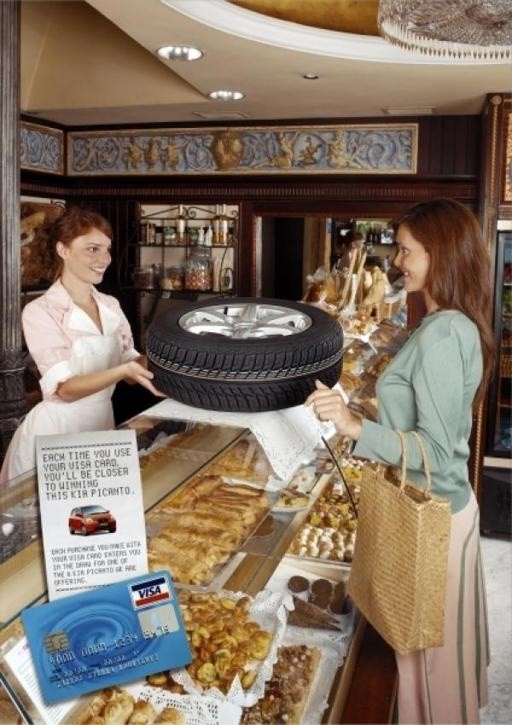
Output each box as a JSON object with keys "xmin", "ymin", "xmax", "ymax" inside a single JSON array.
[{"xmin": 344, "ymin": 429, "xmax": 450, "ymax": 656}]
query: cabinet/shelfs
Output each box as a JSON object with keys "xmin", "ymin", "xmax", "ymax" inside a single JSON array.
[{"xmin": 131, "ymin": 236, "xmax": 235, "ymax": 302}]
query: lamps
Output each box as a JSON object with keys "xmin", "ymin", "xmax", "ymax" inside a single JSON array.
[{"xmin": 373, "ymin": 0, "xmax": 512, "ymax": 67}]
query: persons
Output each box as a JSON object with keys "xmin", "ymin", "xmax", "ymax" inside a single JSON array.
[
  {"xmin": 338, "ymin": 229, "xmax": 365, "ymax": 279},
  {"xmin": 0, "ymin": 208, "xmax": 169, "ymax": 481},
  {"xmin": 303, "ymin": 199, "xmax": 497, "ymax": 724}
]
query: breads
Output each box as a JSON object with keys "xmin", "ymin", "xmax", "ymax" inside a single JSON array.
[{"xmin": 0, "ymin": 267, "xmax": 412, "ymax": 723}]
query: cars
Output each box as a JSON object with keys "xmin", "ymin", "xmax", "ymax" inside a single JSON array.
[{"xmin": 68, "ymin": 504, "xmax": 117, "ymax": 537}]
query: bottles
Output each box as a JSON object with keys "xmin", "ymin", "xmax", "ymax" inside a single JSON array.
[
  {"xmin": 176, "ymin": 205, "xmax": 187, "ymax": 241},
  {"xmin": 185, "ymin": 246, "xmax": 213, "ymax": 291},
  {"xmin": 212, "ymin": 200, "xmax": 230, "ymax": 245},
  {"xmin": 357, "ymin": 223, "xmax": 383, "ymax": 244}
]
